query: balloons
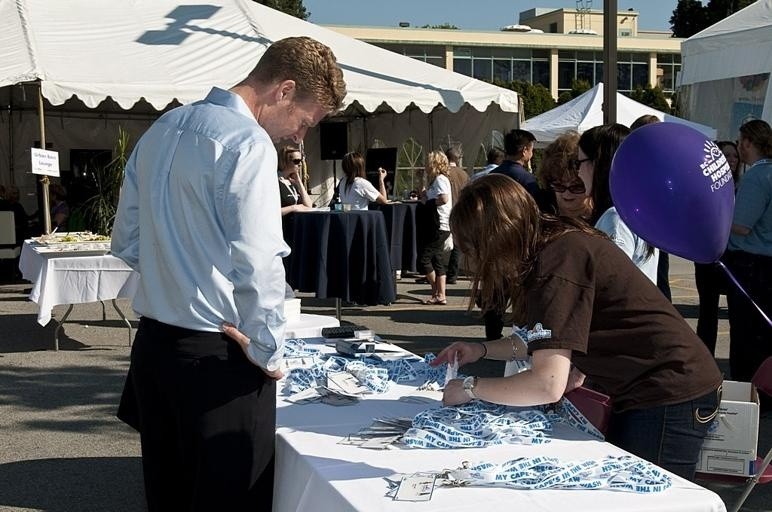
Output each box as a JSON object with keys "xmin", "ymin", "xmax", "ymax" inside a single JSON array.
[{"xmin": 609, "ymin": 121, "xmax": 735, "ymax": 265}]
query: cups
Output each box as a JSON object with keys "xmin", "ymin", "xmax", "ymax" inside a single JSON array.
[
  {"xmin": 335, "ymin": 203, "xmax": 342, "ymax": 211},
  {"xmin": 343, "ymin": 203, "xmax": 351, "ymax": 212}
]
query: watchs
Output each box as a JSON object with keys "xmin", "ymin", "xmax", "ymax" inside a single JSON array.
[{"xmin": 462, "ymin": 376, "xmax": 480, "ymax": 400}]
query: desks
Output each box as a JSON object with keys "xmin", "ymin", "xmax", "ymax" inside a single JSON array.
[
  {"xmin": 366, "ymin": 200, "xmax": 431, "ymax": 277},
  {"xmin": 17, "ymin": 232, "xmax": 139, "ymax": 352},
  {"xmin": 282, "ymin": 210, "xmax": 396, "ymax": 326},
  {"xmin": 271, "ymin": 338, "xmax": 730, "ymax": 510}
]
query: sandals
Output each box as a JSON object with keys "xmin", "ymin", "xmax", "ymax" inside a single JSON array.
[{"xmin": 427, "ymin": 297, "xmax": 447, "ymax": 305}]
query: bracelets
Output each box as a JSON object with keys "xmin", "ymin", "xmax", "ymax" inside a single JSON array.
[
  {"xmin": 476, "ymin": 342, "xmax": 487, "ymax": 359},
  {"xmin": 506, "ymin": 335, "xmax": 517, "ymax": 361}
]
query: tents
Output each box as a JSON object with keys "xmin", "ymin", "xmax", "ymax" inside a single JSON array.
[
  {"xmin": 0, "ymin": 0, "xmax": 532, "ymax": 235},
  {"xmin": 520, "ymin": 83, "xmax": 718, "ymax": 146},
  {"xmin": 678, "ymin": 1, "xmax": 772, "ymax": 143}
]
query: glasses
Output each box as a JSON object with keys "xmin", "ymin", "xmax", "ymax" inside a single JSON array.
[
  {"xmin": 550, "ymin": 182, "xmax": 588, "ymax": 194},
  {"xmin": 290, "ymin": 158, "xmax": 304, "ymax": 164},
  {"xmin": 575, "ymin": 156, "xmax": 593, "ymax": 170},
  {"xmin": 408, "ymin": 194, "xmax": 419, "ymax": 197}
]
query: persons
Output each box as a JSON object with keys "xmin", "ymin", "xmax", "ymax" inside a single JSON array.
[
  {"xmin": 725, "ymin": 120, "xmax": 772, "ymax": 416},
  {"xmin": 414, "ymin": 152, "xmax": 471, "ymax": 284},
  {"xmin": 430, "ymin": 172, "xmax": 723, "ymax": 482},
  {"xmin": 278, "ymin": 145, "xmax": 312, "ymax": 216},
  {"xmin": 474, "ymin": 129, "xmax": 537, "ymax": 339},
  {"xmin": 415, "ymin": 152, "xmax": 454, "ymax": 305},
  {"xmin": 470, "ymin": 147, "xmax": 505, "ymax": 183},
  {"xmin": 110, "ymin": 36, "xmax": 348, "ymax": 512},
  {"xmin": 338, "ymin": 152, "xmax": 388, "ymax": 210},
  {"xmin": 573, "ymin": 124, "xmax": 660, "ymax": 290},
  {"xmin": 695, "ymin": 141, "xmax": 741, "ymax": 361},
  {"xmin": 530, "ymin": 131, "xmax": 597, "ymax": 227}
]
